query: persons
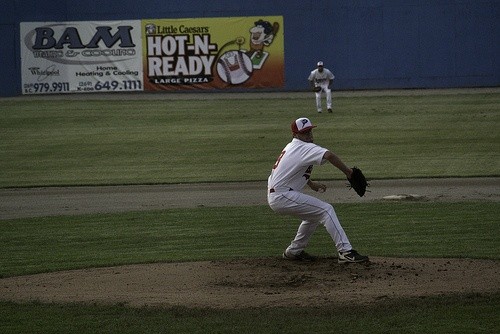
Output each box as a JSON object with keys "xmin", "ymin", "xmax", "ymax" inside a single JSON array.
[
  {"xmin": 267, "ymin": 117, "xmax": 369, "ymax": 264},
  {"xmin": 308, "ymin": 61, "xmax": 335, "ymax": 113}
]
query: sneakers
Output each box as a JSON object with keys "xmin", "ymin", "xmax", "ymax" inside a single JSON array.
[
  {"xmin": 283, "ymin": 250, "xmax": 311, "ymax": 261},
  {"xmin": 337, "ymin": 249, "xmax": 368, "ymax": 264},
  {"xmin": 328, "ymin": 109, "xmax": 332, "ymax": 113},
  {"xmin": 318, "ymin": 111, "xmax": 322, "ymax": 114}
]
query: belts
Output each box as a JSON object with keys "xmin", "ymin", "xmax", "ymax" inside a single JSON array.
[{"xmin": 270, "ymin": 187, "xmax": 294, "ymax": 193}]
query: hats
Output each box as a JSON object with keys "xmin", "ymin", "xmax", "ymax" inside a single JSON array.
[
  {"xmin": 292, "ymin": 117, "xmax": 316, "ymax": 135},
  {"xmin": 317, "ymin": 61, "xmax": 324, "ymax": 66}
]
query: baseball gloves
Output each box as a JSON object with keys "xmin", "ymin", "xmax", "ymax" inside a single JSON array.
[
  {"xmin": 345, "ymin": 166, "xmax": 371, "ymax": 197},
  {"xmin": 314, "ymin": 86, "xmax": 322, "ymax": 92}
]
